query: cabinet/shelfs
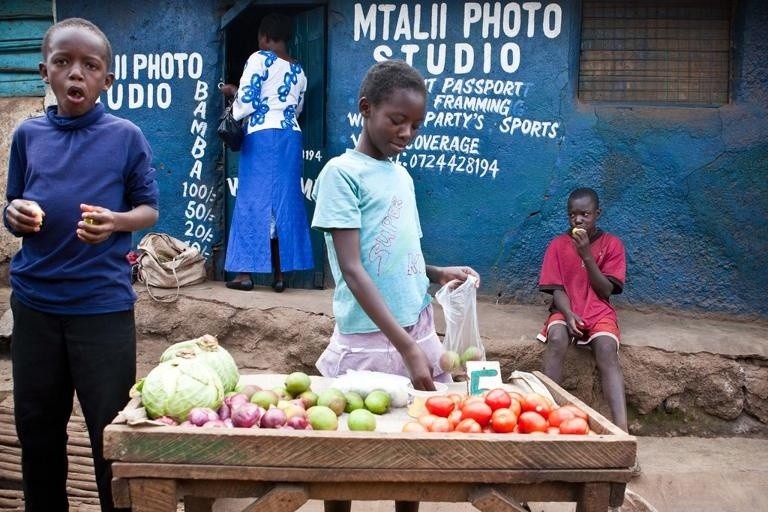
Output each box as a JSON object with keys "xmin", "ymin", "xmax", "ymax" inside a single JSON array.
[{"xmin": 101, "ymin": 374, "xmax": 640, "ymax": 511}]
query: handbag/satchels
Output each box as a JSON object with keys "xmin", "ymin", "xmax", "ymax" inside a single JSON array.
[
  {"xmin": 136, "ymin": 232, "xmax": 207, "ymax": 288},
  {"xmin": 217, "ymin": 107, "xmax": 242, "ymax": 151}
]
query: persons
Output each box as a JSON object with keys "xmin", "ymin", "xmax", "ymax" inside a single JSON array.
[
  {"xmin": 308, "ymin": 59, "xmax": 485, "ymax": 511},
  {"xmin": 2, "ymin": 15, "xmax": 156, "ymax": 511},
  {"xmin": 533, "ymin": 187, "xmax": 631, "ymax": 442},
  {"xmin": 219, "ymin": 13, "xmax": 317, "ymax": 291}
]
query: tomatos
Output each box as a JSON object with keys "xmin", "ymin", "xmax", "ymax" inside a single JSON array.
[{"xmin": 403, "ymin": 389, "xmax": 591, "ymax": 435}]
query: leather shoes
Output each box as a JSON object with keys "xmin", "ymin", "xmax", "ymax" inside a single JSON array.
[
  {"xmin": 272, "ymin": 280, "xmax": 284, "ymax": 291},
  {"xmin": 226, "ymin": 279, "xmax": 253, "ymax": 290}
]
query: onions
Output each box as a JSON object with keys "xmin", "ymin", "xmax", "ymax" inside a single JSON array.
[{"xmin": 153, "ymin": 393, "xmax": 307, "ymax": 437}]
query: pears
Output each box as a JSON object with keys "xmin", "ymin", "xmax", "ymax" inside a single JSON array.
[
  {"xmin": 460, "ymin": 347, "xmax": 482, "ymax": 368},
  {"xmin": 439, "ymin": 351, "xmax": 460, "ymax": 372},
  {"xmin": 225, "ymin": 371, "xmax": 391, "ymax": 431}
]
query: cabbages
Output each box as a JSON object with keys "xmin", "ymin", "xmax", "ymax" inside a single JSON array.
[
  {"xmin": 142, "ymin": 349, "xmax": 224, "ymax": 423},
  {"xmin": 158, "ymin": 335, "xmax": 238, "ymax": 395}
]
